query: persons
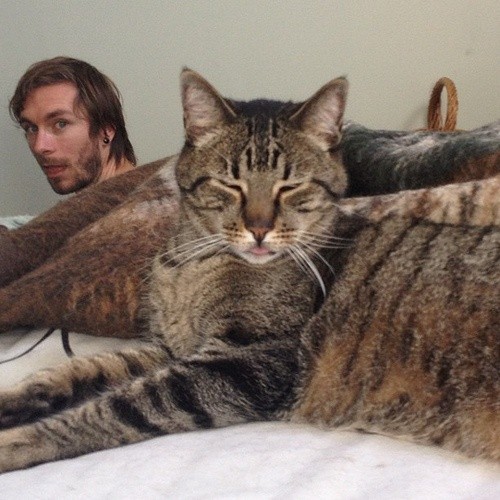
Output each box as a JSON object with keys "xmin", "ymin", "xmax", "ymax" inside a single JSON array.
[{"xmin": 7, "ymin": 56, "xmax": 137, "ymax": 196}]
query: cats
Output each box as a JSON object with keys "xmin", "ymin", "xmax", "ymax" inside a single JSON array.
[{"xmin": 0, "ymin": 64, "xmax": 500, "ymax": 476}]
